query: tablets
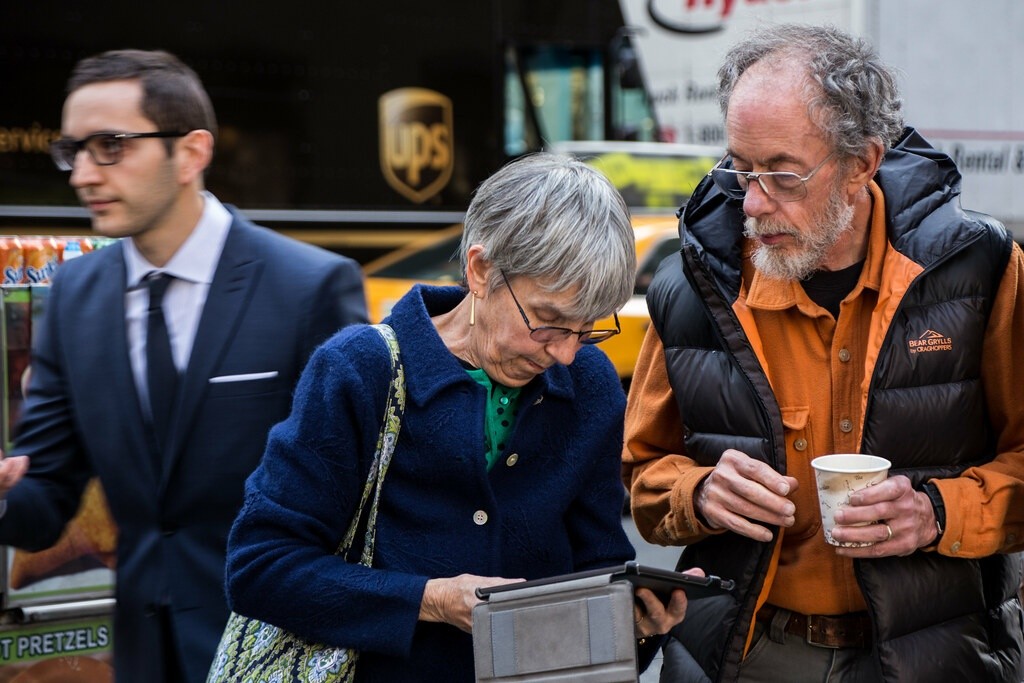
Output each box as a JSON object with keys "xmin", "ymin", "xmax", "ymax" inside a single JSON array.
[{"xmin": 475, "ymin": 560, "xmax": 742, "ymax": 597}]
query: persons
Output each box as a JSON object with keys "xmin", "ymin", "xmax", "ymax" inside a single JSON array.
[
  {"xmin": 0, "ymin": 49, "xmax": 370, "ymax": 683},
  {"xmin": 620, "ymin": 22, "xmax": 1023, "ymax": 683},
  {"xmin": 223, "ymin": 151, "xmax": 707, "ymax": 683}
]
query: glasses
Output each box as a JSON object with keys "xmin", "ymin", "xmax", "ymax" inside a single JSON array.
[
  {"xmin": 708, "ymin": 151, "xmax": 836, "ymax": 202},
  {"xmin": 47, "ymin": 129, "xmax": 188, "ymax": 170},
  {"xmin": 499, "ymin": 267, "xmax": 621, "ymax": 346}
]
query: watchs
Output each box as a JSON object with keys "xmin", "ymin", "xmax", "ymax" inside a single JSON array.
[{"xmin": 915, "ymin": 481, "xmax": 946, "ymax": 552}]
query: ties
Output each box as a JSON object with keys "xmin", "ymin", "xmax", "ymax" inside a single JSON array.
[{"xmin": 141, "ymin": 273, "xmax": 179, "ymax": 460}]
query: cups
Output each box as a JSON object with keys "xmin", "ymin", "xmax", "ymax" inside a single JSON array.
[{"xmin": 811, "ymin": 453, "xmax": 892, "ymax": 549}]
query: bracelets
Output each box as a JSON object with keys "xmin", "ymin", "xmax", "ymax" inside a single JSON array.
[{"xmin": 639, "ymin": 638, "xmax": 648, "ymax": 648}]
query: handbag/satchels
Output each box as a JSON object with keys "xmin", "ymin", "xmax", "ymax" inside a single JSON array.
[{"xmin": 201, "ymin": 326, "xmax": 404, "ymax": 683}]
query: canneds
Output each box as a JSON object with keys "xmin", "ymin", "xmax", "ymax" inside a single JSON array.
[{"xmin": 0, "ymin": 234, "xmax": 118, "ymax": 286}]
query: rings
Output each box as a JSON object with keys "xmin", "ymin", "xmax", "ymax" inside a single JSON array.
[
  {"xmin": 635, "ymin": 615, "xmax": 644, "ymax": 625},
  {"xmin": 884, "ymin": 524, "xmax": 894, "ymax": 541}
]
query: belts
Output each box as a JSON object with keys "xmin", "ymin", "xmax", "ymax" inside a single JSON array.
[{"xmin": 761, "ymin": 606, "xmax": 873, "ymax": 650}]
